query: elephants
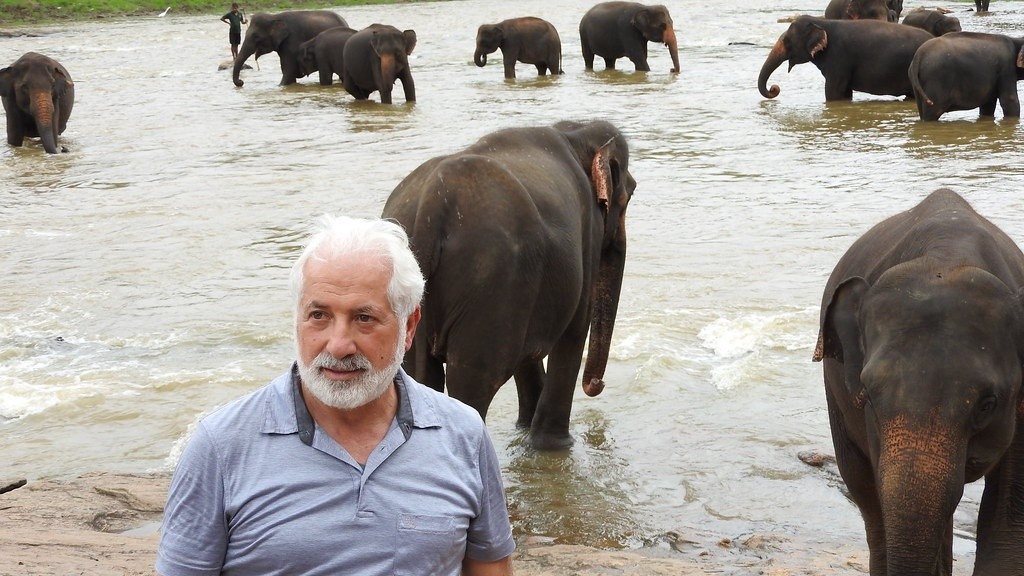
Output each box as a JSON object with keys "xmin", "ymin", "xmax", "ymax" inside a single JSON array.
[
  {"xmin": 579, "ymin": 1, "xmax": 680, "ymax": 73},
  {"xmin": 233, "ymin": 10, "xmax": 416, "ymax": 104},
  {"xmin": 380, "ymin": 121, "xmax": 637, "ymax": 451},
  {"xmin": 474, "ymin": 16, "xmax": 565, "ymax": 79},
  {"xmin": 0, "ymin": 52, "xmax": 74, "ymax": 155},
  {"xmin": 758, "ymin": 0, "xmax": 1024, "ymax": 122},
  {"xmin": 812, "ymin": 188, "xmax": 1023, "ymax": 576}
]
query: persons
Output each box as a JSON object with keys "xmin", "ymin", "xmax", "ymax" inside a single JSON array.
[
  {"xmin": 149, "ymin": 214, "xmax": 515, "ymax": 576},
  {"xmin": 221, "ymin": 3, "xmax": 248, "ymax": 62}
]
